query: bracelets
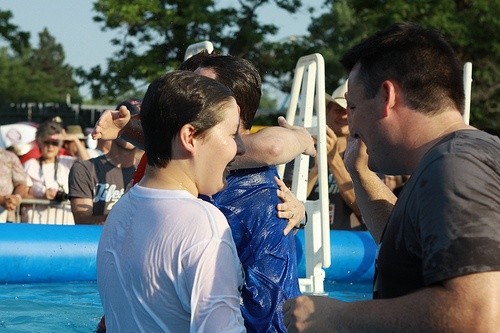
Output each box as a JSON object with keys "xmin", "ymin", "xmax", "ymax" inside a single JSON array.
[{"xmin": 294, "ymin": 210, "xmax": 308, "ymax": 229}]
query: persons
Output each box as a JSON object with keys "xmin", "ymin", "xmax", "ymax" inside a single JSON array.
[
  {"xmin": 96, "ymin": 70, "xmax": 247, "ymax": 333},
  {"xmin": 92, "ymin": 50, "xmax": 317, "ymax": 333},
  {"xmin": 0, "ymin": 122, "xmax": 113, "ymax": 224},
  {"xmin": 284, "ymin": 81, "xmax": 407, "ymax": 230},
  {"xmin": 69, "ymin": 99, "xmax": 145, "ymax": 225},
  {"xmin": 283, "ymin": 22, "xmax": 500, "ymax": 333}
]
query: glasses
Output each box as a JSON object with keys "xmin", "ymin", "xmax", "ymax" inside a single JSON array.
[{"xmin": 42, "ymin": 140, "xmax": 58, "ymax": 146}]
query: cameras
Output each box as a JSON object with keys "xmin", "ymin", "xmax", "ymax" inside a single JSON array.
[{"xmin": 55, "ymin": 191, "xmax": 70, "ymax": 202}]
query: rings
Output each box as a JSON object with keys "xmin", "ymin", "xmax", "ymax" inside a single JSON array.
[{"xmin": 290, "ymin": 210, "xmax": 294, "ymax": 218}]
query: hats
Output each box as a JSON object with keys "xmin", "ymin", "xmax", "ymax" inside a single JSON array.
[
  {"xmin": 314, "ymin": 78, "xmax": 348, "ymax": 114},
  {"xmin": 114, "ymin": 97, "xmax": 143, "ymax": 117},
  {"xmin": 66, "ymin": 124, "xmax": 89, "ymax": 140}
]
